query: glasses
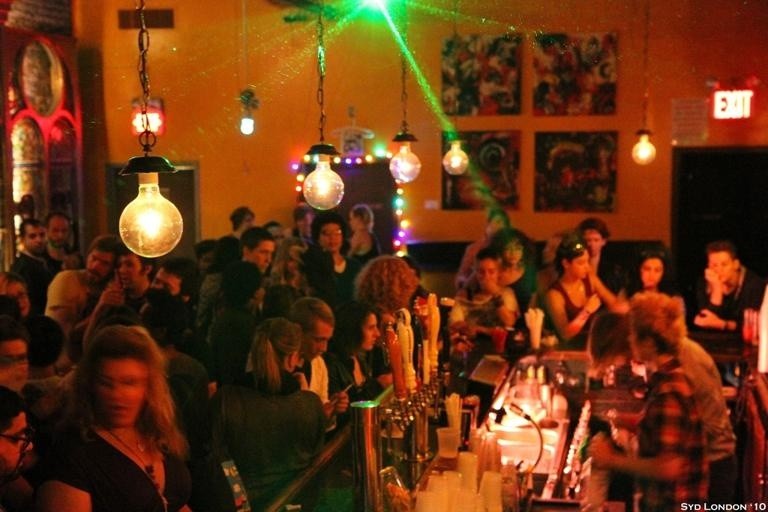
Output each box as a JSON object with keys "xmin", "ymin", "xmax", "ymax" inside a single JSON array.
[
  {"xmin": 324, "ymin": 228, "xmax": 342, "ymax": 234},
  {"xmin": 1, "ymin": 354, "xmax": 32, "ymax": 367},
  {"xmin": 2, "ymin": 422, "xmax": 37, "ymax": 452},
  {"xmin": 505, "ymin": 245, "xmax": 525, "ymax": 251},
  {"xmin": 573, "ymin": 242, "xmax": 584, "ymax": 254}
]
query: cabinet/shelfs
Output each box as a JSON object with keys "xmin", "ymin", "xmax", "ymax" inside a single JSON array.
[
  {"xmin": 528, "ymin": 29, "xmax": 620, "ymax": 117},
  {"xmin": 440, "ymin": 128, "xmax": 521, "ymax": 213},
  {"xmin": 440, "ymin": 31, "xmax": 522, "ymax": 118},
  {"xmin": 532, "ymin": 130, "xmax": 620, "ymax": 215}
]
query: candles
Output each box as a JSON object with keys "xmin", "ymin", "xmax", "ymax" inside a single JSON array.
[{"xmin": 407, "ymin": 448, "xmax": 507, "ymax": 512}]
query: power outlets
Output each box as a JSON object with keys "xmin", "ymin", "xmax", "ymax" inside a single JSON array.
[{"xmin": 424, "ymin": 200, "xmax": 439, "ymax": 211}]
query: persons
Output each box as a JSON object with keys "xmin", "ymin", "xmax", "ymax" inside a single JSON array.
[
  {"xmin": 45, "ymin": 235, "xmax": 123, "ymax": 369},
  {"xmin": 456, "ymin": 208, "xmax": 510, "ymax": 289},
  {"xmin": 206, "ymin": 318, "xmax": 326, "ymax": 511},
  {"xmin": 0, "ymin": 385, "xmax": 36, "ymax": 512},
  {"xmin": 545, "ymin": 240, "xmax": 630, "ymax": 350},
  {"xmin": 694, "ymin": 240, "xmax": 764, "ymax": 333},
  {"xmin": 1, "ymin": 272, "xmax": 33, "ymax": 320},
  {"xmin": 348, "ymin": 204, "xmax": 380, "ymax": 262},
  {"xmin": 310, "ymin": 211, "xmax": 363, "ymax": 308},
  {"xmin": 324, "ymin": 301, "xmax": 394, "ymax": 425},
  {"xmin": 463, "ymin": 228, "xmax": 538, "ymax": 316},
  {"xmin": 286, "ymin": 296, "xmax": 350, "ymax": 442},
  {"xmin": 30, "ymin": 317, "xmax": 75, "ymax": 424},
  {"xmin": 588, "ymin": 292, "xmax": 708, "ymax": 512},
  {"xmin": 589, "ymin": 314, "xmax": 737, "ymax": 512},
  {"xmin": 618, "ymin": 248, "xmax": 693, "ymax": 329},
  {"xmin": 0, "ymin": 312, "xmax": 31, "ymax": 390},
  {"xmin": 45, "ymin": 213, "xmax": 82, "ymax": 270},
  {"xmin": 454, "ymin": 249, "xmax": 519, "ymax": 338},
  {"xmin": 150, "ymin": 204, "xmax": 314, "ymax": 364},
  {"xmin": 83, "ymin": 251, "xmax": 187, "ymax": 363},
  {"xmin": 541, "ymin": 216, "xmax": 630, "ymax": 329},
  {"xmin": 354, "ymin": 257, "xmax": 418, "ymax": 369},
  {"xmin": 5, "ymin": 218, "xmax": 60, "ymax": 316},
  {"xmin": 37, "ymin": 325, "xmax": 193, "ymax": 512}
]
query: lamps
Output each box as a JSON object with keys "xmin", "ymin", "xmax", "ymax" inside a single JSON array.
[
  {"xmin": 633, "ymin": 2, "xmax": 657, "ymax": 164},
  {"xmin": 119, "ymin": 2, "xmax": 183, "ymax": 259},
  {"xmin": 301, "ymin": 1, "xmax": 347, "ymax": 209},
  {"xmin": 388, "ymin": 30, "xmax": 422, "ymax": 184},
  {"xmin": 237, "ymin": 0, "xmax": 264, "ymax": 137},
  {"xmin": 441, "ymin": 7, "xmax": 470, "ymax": 174}
]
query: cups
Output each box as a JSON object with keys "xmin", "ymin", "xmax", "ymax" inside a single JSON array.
[
  {"xmin": 458, "ymin": 489, "xmax": 476, "ymax": 512},
  {"xmin": 427, "ymin": 475, "xmax": 448, "ymax": 512},
  {"xmin": 479, "ymin": 470, "xmax": 502, "ymax": 512},
  {"xmin": 443, "ymin": 470, "xmax": 462, "ymax": 512},
  {"xmin": 458, "ymin": 452, "xmax": 478, "ymax": 489},
  {"xmin": 435, "ymin": 427, "xmax": 462, "ymax": 459},
  {"xmin": 416, "ymin": 491, "xmax": 439, "ymax": 512},
  {"xmin": 479, "ymin": 432, "xmax": 500, "ymax": 471},
  {"xmin": 476, "ymin": 494, "xmax": 487, "ymax": 512}
]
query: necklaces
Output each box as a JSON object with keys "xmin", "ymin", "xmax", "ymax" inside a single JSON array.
[{"xmin": 104, "ymin": 423, "xmax": 162, "ymax": 490}]
